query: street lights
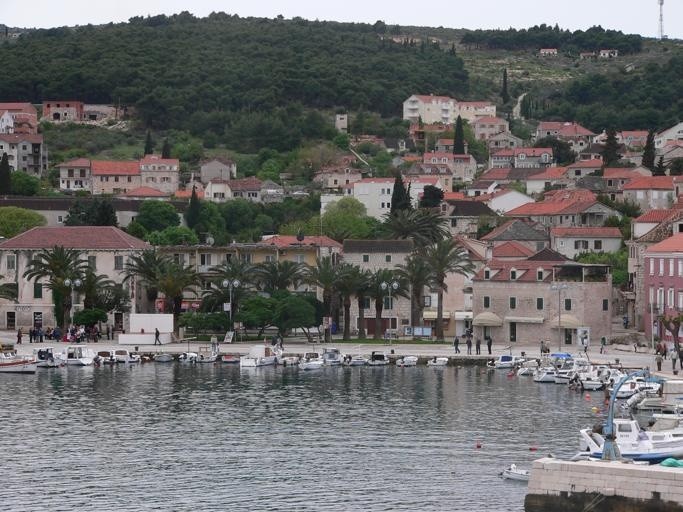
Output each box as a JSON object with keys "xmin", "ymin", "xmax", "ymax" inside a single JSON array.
[
  {"xmin": 380, "ymin": 281, "xmax": 399, "ymax": 344},
  {"xmin": 64, "ymin": 279, "xmax": 81, "ymax": 326},
  {"xmin": 222, "ymin": 278, "xmax": 239, "ymax": 332},
  {"xmin": 551, "ymin": 285, "xmax": 568, "ymax": 352}
]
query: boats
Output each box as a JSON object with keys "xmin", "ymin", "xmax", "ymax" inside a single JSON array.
[
  {"xmin": 396, "ymin": 355, "xmax": 419, "ymax": 368},
  {"xmin": 221, "ymin": 352, "xmax": 240, "ymax": 363},
  {"xmin": 0, "ymin": 352, "xmax": 38, "ymax": 374},
  {"xmin": 25, "ymin": 347, "xmax": 149, "ymax": 368},
  {"xmin": 152, "ymin": 348, "xmax": 176, "ymax": 362},
  {"xmin": 179, "ymin": 346, "xmax": 219, "ymax": 363},
  {"xmin": 426, "ymin": 356, "xmax": 449, "ymax": 367},
  {"xmin": 239, "ymin": 344, "xmax": 390, "ymax": 368},
  {"xmin": 486, "ymin": 352, "xmax": 683, "ymax": 481}
]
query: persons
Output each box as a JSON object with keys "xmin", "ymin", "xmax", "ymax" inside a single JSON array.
[
  {"xmin": 487, "ymin": 336, "xmax": 492, "ymax": 354},
  {"xmin": 476, "ymin": 337, "xmax": 481, "ymax": 354},
  {"xmin": 599, "ymin": 336, "xmax": 606, "ymax": 354},
  {"xmin": 569, "ymin": 373, "xmax": 584, "ymax": 394},
  {"xmin": 540, "ymin": 340, "xmax": 549, "ymax": 356},
  {"xmin": 466, "ymin": 336, "xmax": 471, "ymax": 355},
  {"xmin": 653, "ymin": 340, "xmax": 683, "ymax": 371},
  {"xmin": 453, "ymin": 336, "xmax": 460, "ymax": 353},
  {"xmin": 584, "ymin": 337, "xmax": 587, "ymax": 352},
  {"xmin": 28, "ymin": 325, "xmax": 102, "ymax": 342},
  {"xmin": 155, "ymin": 328, "xmax": 161, "ymax": 345},
  {"xmin": 17, "ymin": 328, "xmax": 22, "ymax": 344},
  {"xmin": 601, "ymin": 384, "xmax": 610, "ymax": 400},
  {"xmin": 106, "ymin": 325, "xmax": 110, "ymax": 340},
  {"xmin": 111, "ymin": 325, "xmax": 114, "ymax": 340},
  {"xmin": 271, "ymin": 333, "xmax": 283, "ymax": 348}
]
